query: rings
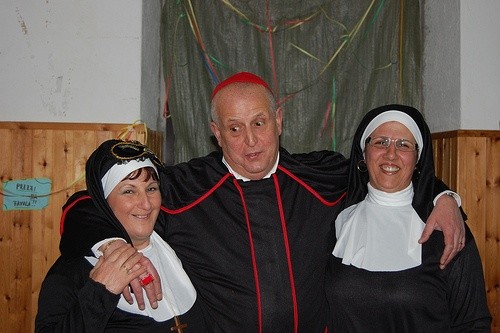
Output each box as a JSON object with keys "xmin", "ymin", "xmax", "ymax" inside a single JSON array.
[
  {"xmin": 458, "ymin": 241, "xmax": 462, "ymax": 244},
  {"xmin": 140, "ymin": 273, "xmax": 154, "ymax": 287},
  {"xmin": 122, "ymin": 264, "xmax": 129, "ymax": 271}
]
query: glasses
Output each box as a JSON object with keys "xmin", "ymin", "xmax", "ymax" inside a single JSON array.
[
  {"xmin": 366, "ymin": 136, "xmax": 418, "ymax": 152},
  {"xmin": 109, "ymin": 141, "xmax": 164, "ymax": 167}
]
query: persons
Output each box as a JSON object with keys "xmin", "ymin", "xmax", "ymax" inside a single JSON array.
[
  {"xmin": 59, "ymin": 73, "xmax": 468, "ymax": 333},
  {"xmin": 33, "ymin": 141, "xmax": 206, "ymax": 333},
  {"xmin": 323, "ymin": 103, "xmax": 493, "ymax": 333}
]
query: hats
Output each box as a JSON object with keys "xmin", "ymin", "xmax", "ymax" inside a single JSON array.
[{"xmin": 211, "ymin": 72, "xmax": 273, "ymax": 94}]
